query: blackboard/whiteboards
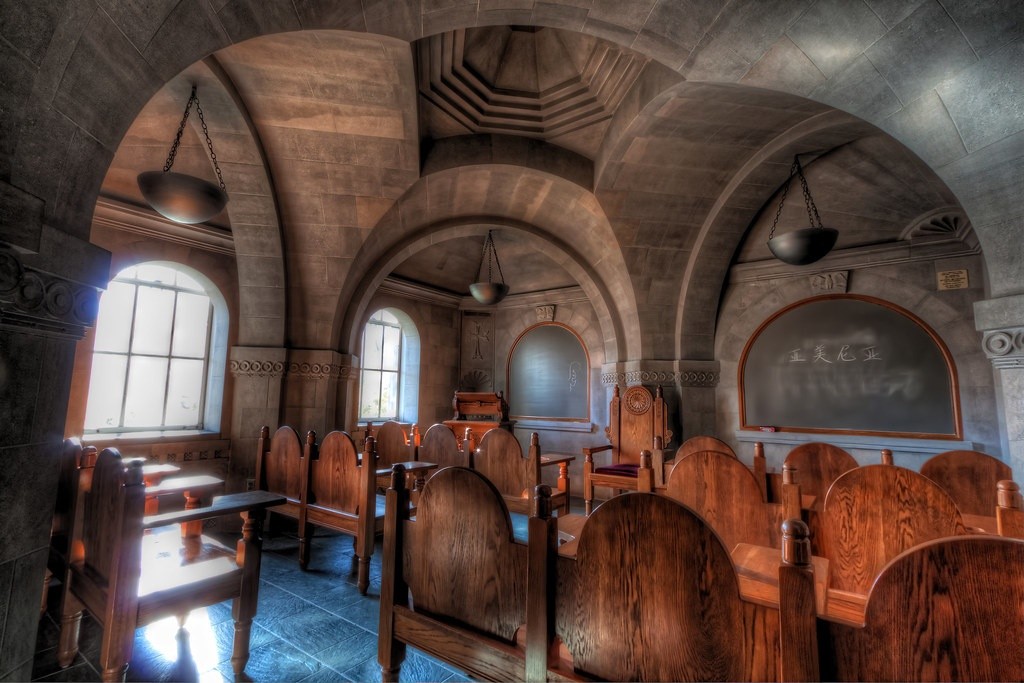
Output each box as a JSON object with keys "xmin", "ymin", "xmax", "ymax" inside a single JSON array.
[
  {"xmin": 737, "ymin": 293, "xmax": 964, "ymax": 441},
  {"xmin": 505, "ymin": 321, "xmax": 591, "ymax": 423}
]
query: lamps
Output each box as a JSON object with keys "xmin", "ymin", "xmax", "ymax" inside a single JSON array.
[
  {"xmin": 136, "ymin": 86, "xmax": 229, "ymax": 224},
  {"xmin": 766, "ymin": 155, "xmax": 839, "ymax": 266},
  {"xmin": 468, "ymin": 230, "xmax": 510, "ymax": 306}
]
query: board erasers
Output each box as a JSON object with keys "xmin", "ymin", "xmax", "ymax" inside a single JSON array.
[{"xmin": 759, "ymin": 426, "xmax": 775, "ymax": 432}]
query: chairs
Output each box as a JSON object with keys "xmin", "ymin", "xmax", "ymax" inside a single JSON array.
[{"xmin": 40, "ymin": 383, "xmax": 1024, "ymax": 683}]
